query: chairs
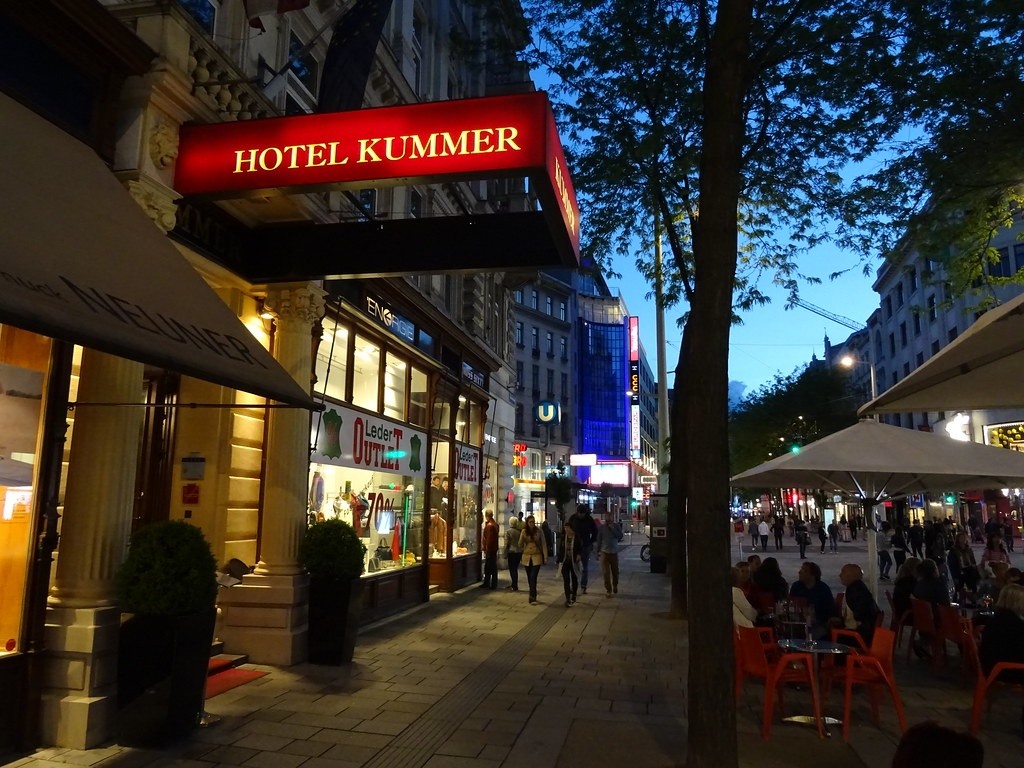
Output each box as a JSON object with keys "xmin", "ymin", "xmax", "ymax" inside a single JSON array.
[{"xmin": 734, "ymin": 590, "xmax": 1024, "ymax": 741}]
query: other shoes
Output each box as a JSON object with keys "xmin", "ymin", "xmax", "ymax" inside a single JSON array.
[
  {"xmin": 528, "ymin": 593, "xmax": 539, "ymax": 606},
  {"xmin": 613, "ymin": 582, "xmax": 618, "ymax": 593},
  {"xmin": 564, "ymin": 598, "xmax": 576, "ymax": 607},
  {"xmin": 490, "ymin": 585, "xmax": 498, "ymax": 590},
  {"xmin": 582, "ymin": 586, "xmax": 588, "ymax": 594},
  {"xmin": 606, "ymin": 590, "xmax": 612, "ymax": 599},
  {"xmin": 478, "ymin": 583, "xmax": 490, "ymax": 589},
  {"xmin": 506, "ymin": 585, "xmax": 518, "ymax": 592}
]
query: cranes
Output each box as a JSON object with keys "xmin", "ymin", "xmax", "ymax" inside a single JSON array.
[{"xmin": 787, "ymin": 295, "xmax": 866, "ymax": 331}]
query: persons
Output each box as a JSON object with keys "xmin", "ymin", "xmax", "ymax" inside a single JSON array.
[{"xmin": 431, "ymin": 475, "xmax": 1023, "ymax": 693}]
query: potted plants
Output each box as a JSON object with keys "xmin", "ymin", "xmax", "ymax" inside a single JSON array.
[
  {"xmin": 113, "ymin": 518, "xmax": 219, "ymax": 750},
  {"xmin": 298, "ymin": 518, "xmax": 368, "ymax": 663}
]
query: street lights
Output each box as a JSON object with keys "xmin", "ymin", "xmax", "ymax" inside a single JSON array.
[{"xmin": 840, "ymin": 356, "xmax": 880, "ymax": 422}]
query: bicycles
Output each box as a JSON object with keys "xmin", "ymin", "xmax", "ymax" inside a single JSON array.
[{"xmin": 640, "ymin": 535, "xmax": 651, "ymax": 562}]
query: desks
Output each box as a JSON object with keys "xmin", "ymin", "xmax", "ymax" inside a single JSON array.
[
  {"xmin": 780, "ymin": 638, "xmax": 848, "ymax": 735},
  {"xmin": 768, "ymin": 611, "xmax": 815, "ymax": 640}
]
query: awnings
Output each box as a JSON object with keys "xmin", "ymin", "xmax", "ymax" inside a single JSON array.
[{"xmin": 1, "ymin": 90, "xmax": 327, "ymax": 416}]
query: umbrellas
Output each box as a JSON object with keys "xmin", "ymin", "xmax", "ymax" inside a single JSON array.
[{"xmin": 729, "ymin": 415, "xmax": 1024, "ymax": 607}]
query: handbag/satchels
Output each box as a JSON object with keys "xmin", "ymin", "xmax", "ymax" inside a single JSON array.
[{"xmin": 806, "ymin": 537, "xmax": 812, "ymax": 545}]
